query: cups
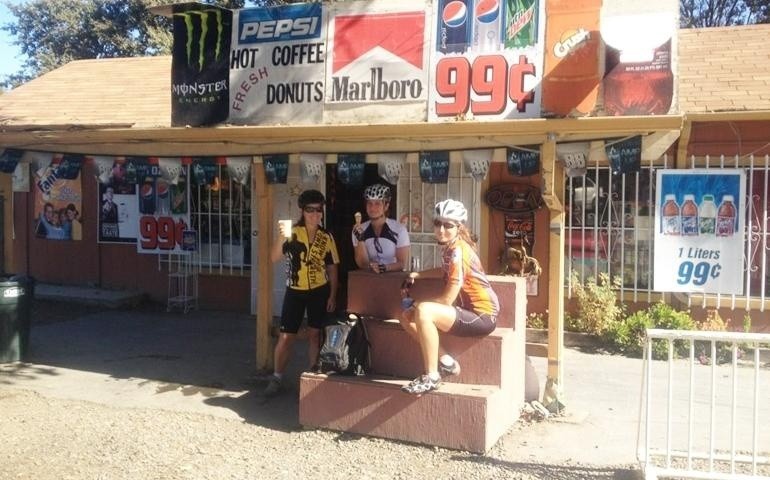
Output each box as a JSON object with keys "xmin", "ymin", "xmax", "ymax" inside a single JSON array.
[{"xmin": 278, "ymin": 219, "xmax": 293, "ymax": 239}]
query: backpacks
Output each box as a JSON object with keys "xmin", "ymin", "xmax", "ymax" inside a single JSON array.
[{"xmin": 313, "ymin": 313, "xmax": 372, "ymax": 376}]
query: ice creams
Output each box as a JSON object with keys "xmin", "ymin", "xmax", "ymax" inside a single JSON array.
[{"xmin": 354, "ymin": 212, "xmax": 362, "ymax": 227}]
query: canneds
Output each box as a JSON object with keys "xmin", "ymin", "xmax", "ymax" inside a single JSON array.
[
  {"xmin": 170, "ymin": 176, "xmax": 187, "ymax": 214},
  {"xmin": 138, "ymin": 176, "xmax": 155, "ymax": 214},
  {"xmin": 472, "ymin": 0, "xmax": 503, "ymax": 46},
  {"xmin": 155, "ymin": 177, "xmax": 170, "ymax": 215},
  {"xmin": 440, "ymin": 1, "xmax": 470, "ymax": 53},
  {"xmin": 504, "ymin": 1, "xmax": 537, "ymax": 48}
]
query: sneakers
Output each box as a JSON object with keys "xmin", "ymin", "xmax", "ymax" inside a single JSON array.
[
  {"xmin": 438, "ymin": 362, "xmax": 460, "ymax": 378},
  {"xmin": 264, "ymin": 376, "xmax": 287, "ymax": 396},
  {"xmin": 400, "ymin": 374, "xmax": 442, "ymax": 395}
]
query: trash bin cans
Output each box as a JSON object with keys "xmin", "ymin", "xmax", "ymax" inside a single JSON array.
[{"xmin": 0, "ymin": 273, "xmax": 36, "ymax": 371}]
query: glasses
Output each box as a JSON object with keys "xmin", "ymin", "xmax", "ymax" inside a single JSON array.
[
  {"xmin": 434, "ymin": 220, "xmax": 457, "ymax": 229},
  {"xmin": 305, "ymin": 207, "xmax": 321, "ymax": 212}
]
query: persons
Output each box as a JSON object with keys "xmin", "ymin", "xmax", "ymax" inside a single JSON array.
[
  {"xmin": 34, "ymin": 201, "xmax": 83, "ymax": 242},
  {"xmin": 400, "ymin": 199, "xmax": 500, "ymax": 394},
  {"xmin": 262, "ymin": 190, "xmax": 340, "ymax": 397},
  {"xmin": 101, "ymin": 187, "xmax": 120, "ymax": 238},
  {"xmin": 351, "ymin": 183, "xmax": 410, "ymax": 274}
]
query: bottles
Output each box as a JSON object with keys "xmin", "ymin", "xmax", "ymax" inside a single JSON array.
[
  {"xmin": 542, "ymin": 0, "xmax": 602, "ymax": 118},
  {"xmin": 603, "ymin": 38, "xmax": 674, "ymax": 116},
  {"xmin": 661, "ymin": 193, "xmax": 737, "ymax": 238},
  {"xmin": 503, "ymin": 189, "xmax": 535, "ymax": 275}
]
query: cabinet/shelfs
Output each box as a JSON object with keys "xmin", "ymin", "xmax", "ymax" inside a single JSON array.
[{"xmin": 165, "ymin": 245, "xmax": 199, "ymax": 314}]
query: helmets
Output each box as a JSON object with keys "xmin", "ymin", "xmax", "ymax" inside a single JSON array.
[
  {"xmin": 434, "ymin": 199, "xmax": 467, "ymax": 221},
  {"xmin": 364, "ymin": 184, "xmax": 390, "ymax": 200},
  {"xmin": 298, "ymin": 190, "xmax": 324, "ymax": 207}
]
